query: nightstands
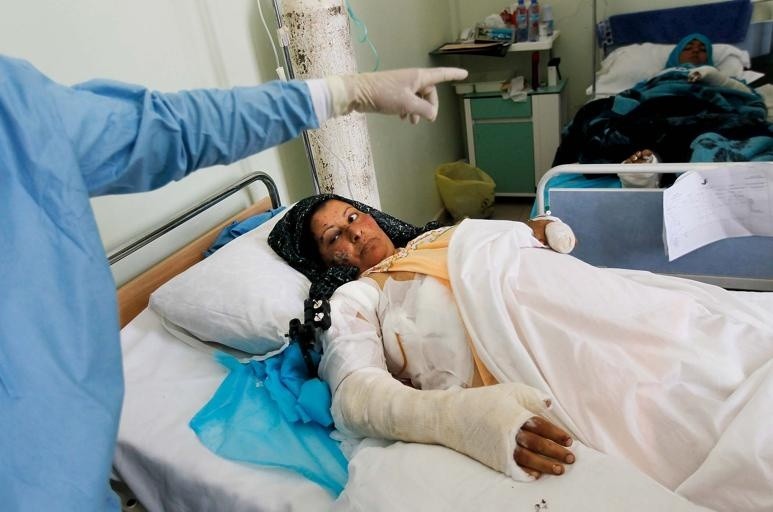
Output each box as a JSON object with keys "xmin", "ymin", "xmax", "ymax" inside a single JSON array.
[{"xmin": 430, "ymin": 30, "xmax": 568, "ymax": 197}]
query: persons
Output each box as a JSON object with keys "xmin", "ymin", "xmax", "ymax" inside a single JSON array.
[
  {"xmin": 268, "ymin": 193, "xmax": 579, "ymax": 485},
  {"xmin": 613, "ymin": 33, "xmax": 752, "ymax": 188},
  {"xmin": 0, "ymin": 51, "xmax": 469, "ymax": 512}
]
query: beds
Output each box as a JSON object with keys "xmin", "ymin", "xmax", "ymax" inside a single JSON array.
[
  {"xmin": 529, "ymin": 0, "xmax": 773, "ymax": 291},
  {"xmin": 105, "ymin": 172, "xmax": 772, "ymax": 512}
]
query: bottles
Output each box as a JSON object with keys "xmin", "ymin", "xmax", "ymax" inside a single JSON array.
[
  {"xmin": 525, "ymin": 1, "xmax": 541, "ymax": 42},
  {"xmin": 514, "ymin": 0, "xmax": 528, "ymax": 43},
  {"xmin": 539, "ymin": 4, "xmax": 554, "ymax": 36}
]
